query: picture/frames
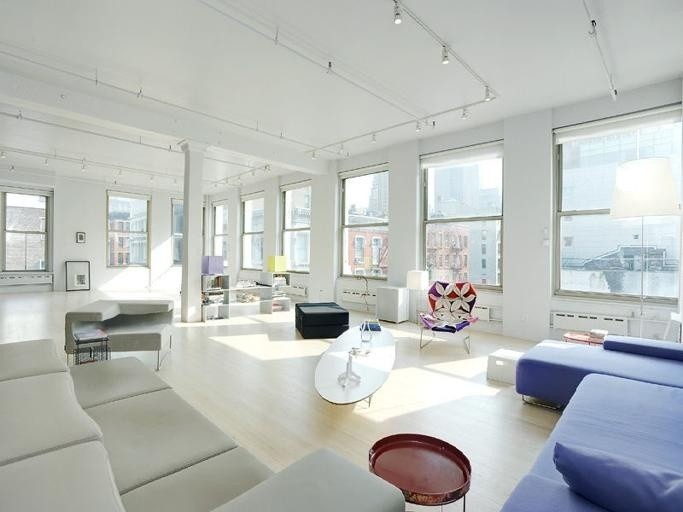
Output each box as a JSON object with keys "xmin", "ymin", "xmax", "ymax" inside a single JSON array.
[
  {"xmin": 64, "ymin": 261, "xmax": 90, "ymax": 292},
  {"xmin": 75, "ymin": 231, "xmax": 86, "ymax": 244}
]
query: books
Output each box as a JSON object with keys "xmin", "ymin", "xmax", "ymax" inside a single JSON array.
[
  {"xmin": 206, "ymin": 276, "xmax": 222, "ymax": 289},
  {"xmin": 73, "ymin": 328, "xmax": 108, "ymax": 341},
  {"xmin": 359, "ymin": 318, "xmax": 382, "ymax": 332},
  {"xmin": 586, "ymin": 329, "xmax": 608, "ymax": 344}
]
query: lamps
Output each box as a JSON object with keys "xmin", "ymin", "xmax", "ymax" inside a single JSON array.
[
  {"xmin": 611, "ymin": 157, "xmax": 682, "ymax": 318},
  {"xmin": 406, "ymin": 271, "xmax": 430, "ymax": 326}
]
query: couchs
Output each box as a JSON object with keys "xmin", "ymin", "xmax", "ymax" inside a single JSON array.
[
  {"xmin": 495, "ymin": 334, "xmax": 683, "ymax": 511},
  {"xmin": 0, "ymin": 340, "xmax": 406, "ymax": 512},
  {"xmin": 65, "ymin": 299, "xmax": 173, "ymax": 370}
]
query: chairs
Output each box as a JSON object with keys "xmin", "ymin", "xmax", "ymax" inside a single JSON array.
[{"xmin": 417, "ymin": 281, "xmax": 477, "ymax": 354}]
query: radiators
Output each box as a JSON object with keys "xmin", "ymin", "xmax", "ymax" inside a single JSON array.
[
  {"xmin": 551, "ymin": 311, "xmax": 630, "ymax": 337},
  {"xmin": 342, "ymin": 289, "xmax": 376, "ymax": 306},
  {"xmin": 474, "ymin": 305, "xmax": 492, "ymax": 323},
  {"xmin": 277, "ymin": 284, "xmax": 307, "ymax": 296}
]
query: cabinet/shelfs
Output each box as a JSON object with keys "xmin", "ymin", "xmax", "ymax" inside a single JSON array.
[{"xmin": 201, "ymin": 272, "xmax": 289, "ymax": 320}]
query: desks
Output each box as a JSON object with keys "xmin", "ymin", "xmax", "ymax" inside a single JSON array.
[
  {"xmin": 376, "ymin": 285, "xmax": 409, "ymax": 324},
  {"xmin": 369, "ymin": 434, "xmax": 471, "ymax": 512},
  {"xmin": 314, "ymin": 324, "xmax": 396, "ymax": 405},
  {"xmin": 563, "ymin": 331, "xmax": 613, "ymax": 345},
  {"xmin": 72, "ymin": 327, "xmax": 109, "ymax": 364},
  {"xmin": 296, "ymin": 302, "xmax": 348, "ymax": 340}
]
